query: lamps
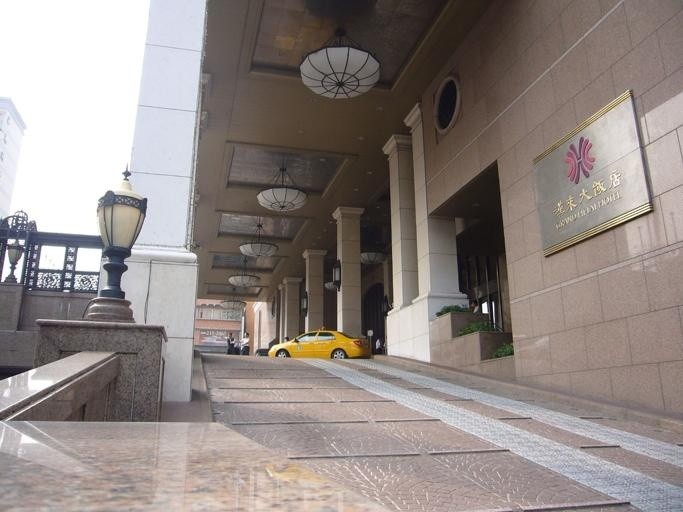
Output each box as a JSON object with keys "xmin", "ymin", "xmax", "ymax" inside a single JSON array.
[
  {"xmin": 97, "ymin": 162, "xmax": 147, "ymax": 300},
  {"xmin": 299, "ymin": 27, "xmax": 381, "ymax": 100},
  {"xmin": 6, "ymin": 233, "xmax": 25, "ymax": 275},
  {"xmin": 219, "ymin": 217, "xmax": 387, "ymax": 310},
  {"xmin": 257, "ymin": 167, "xmax": 309, "ymax": 212}
]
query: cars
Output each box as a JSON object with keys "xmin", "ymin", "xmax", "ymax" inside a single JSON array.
[{"xmin": 267, "ymin": 330, "xmax": 371, "ymax": 359}]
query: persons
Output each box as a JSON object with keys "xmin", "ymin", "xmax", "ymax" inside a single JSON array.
[
  {"xmin": 224, "ymin": 332, "xmax": 234, "ymax": 355},
  {"xmin": 283, "ymin": 337, "xmax": 289, "ymax": 342},
  {"xmin": 374, "ymin": 338, "xmax": 383, "ymax": 354},
  {"xmin": 237, "ymin": 332, "xmax": 250, "ymax": 355}
]
